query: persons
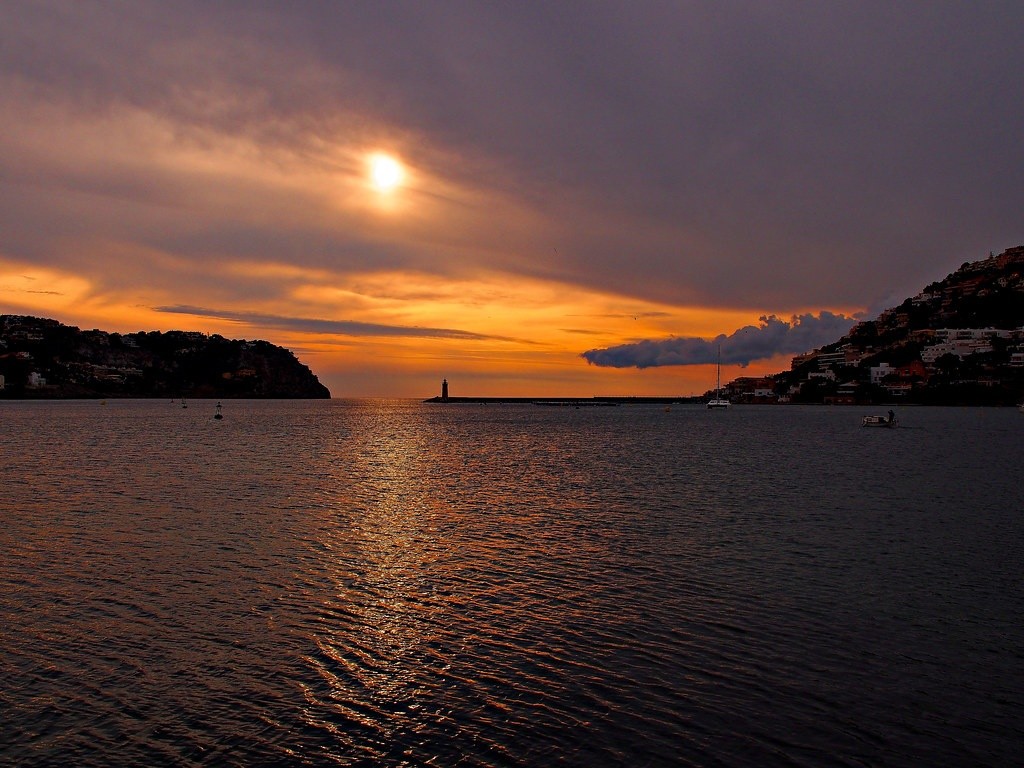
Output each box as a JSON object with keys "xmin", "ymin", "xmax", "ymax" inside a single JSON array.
[{"xmin": 888, "ymin": 409, "xmax": 894, "ymax": 421}]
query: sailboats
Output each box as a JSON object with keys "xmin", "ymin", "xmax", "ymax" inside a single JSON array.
[{"xmin": 706, "ymin": 344, "xmax": 732, "ymax": 410}]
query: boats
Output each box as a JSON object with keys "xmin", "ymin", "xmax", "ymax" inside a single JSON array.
[{"xmin": 861, "ymin": 414, "xmax": 901, "ymax": 429}]
